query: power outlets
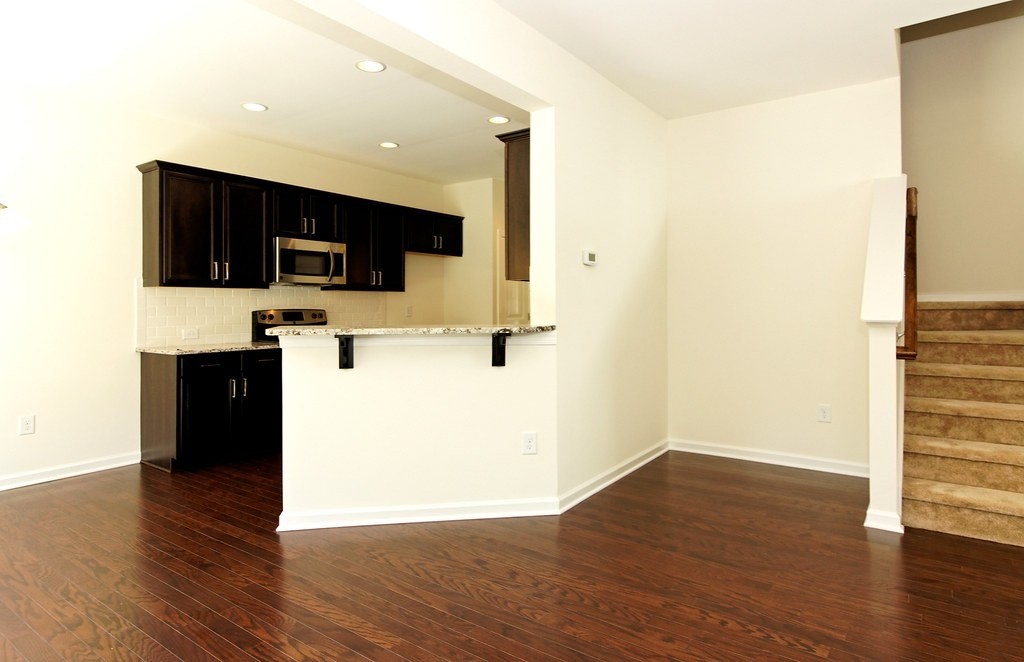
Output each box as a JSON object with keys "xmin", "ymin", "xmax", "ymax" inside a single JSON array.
[
  {"xmin": 182, "ymin": 328, "xmax": 199, "ymax": 340},
  {"xmin": 18, "ymin": 414, "xmax": 35, "ymax": 435}
]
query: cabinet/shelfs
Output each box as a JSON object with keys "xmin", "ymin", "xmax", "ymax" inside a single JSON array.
[
  {"xmin": 136, "ymin": 160, "xmax": 465, "ymax": 292},
  {"xmin": 136, "ymin": 342, "xmax": 282, "ymax": 472},
  {"xmin": 495, "ymin": 126, "xmax": 530, "ymax": 281}
]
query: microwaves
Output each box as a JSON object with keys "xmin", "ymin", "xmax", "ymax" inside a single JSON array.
[{"xmin": 275, "ymin": 236, "xmax": 347, "ymax": 285}]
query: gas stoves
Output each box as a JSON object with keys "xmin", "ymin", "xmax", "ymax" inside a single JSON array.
[{"xmin": 251, "ymin": 309, "xmax": 327, "ymax": 344}]
